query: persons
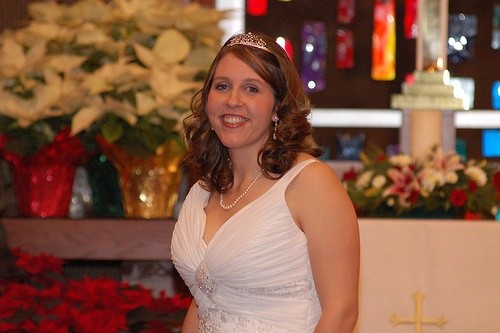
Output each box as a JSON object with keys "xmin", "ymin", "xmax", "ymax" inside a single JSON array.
[{"xmin": 169, "ymin": 31, "xmax": 361, "ymax": 333}]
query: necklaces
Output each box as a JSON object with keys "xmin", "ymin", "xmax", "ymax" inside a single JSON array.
[{"xmin": 220, "ymin": 161, "xmax": 265, "ymax": 209}]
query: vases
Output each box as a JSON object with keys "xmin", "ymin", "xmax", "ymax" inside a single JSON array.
[
  {"xmin": 0, "ymin": 127, "xmax": 87, "ymax": 216},
  {"xmin": 94, "ymin": 132, "xmax": 184, "ymax": 219}
]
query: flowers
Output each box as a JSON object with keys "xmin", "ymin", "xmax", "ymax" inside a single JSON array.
[
  {"xmin": 0, "ymin": 0, "xmax": 236, "ymax": 155},
  {"xmin": 342, "ymin": 144, "xmax": 500, "ymax": 220},
  {"xmin": 0, "ymin": 246, "xmax": 194, "ymax": 333}
]
